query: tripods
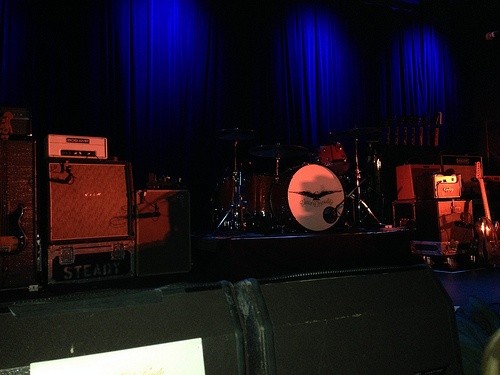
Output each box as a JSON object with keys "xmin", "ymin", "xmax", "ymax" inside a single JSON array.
[
  {"xmin": 211, "ymin": 139, "xmax": 264, "ymax": 237},
  {"xmin": 345, "ymin": 139, "xmax": 384, "ymax": 230}
]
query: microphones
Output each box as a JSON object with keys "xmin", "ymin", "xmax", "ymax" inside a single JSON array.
[{"xmin": 455, "ymin": 220, "xmax": 472, "ymax": 228}]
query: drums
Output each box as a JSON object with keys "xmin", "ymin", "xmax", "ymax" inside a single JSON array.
[
  {"xmin": 313, "ymin": 142, "xmax": 350, "ymax": 176},
  {"xmin": 243, "ymin": 172, "xmax": 276, "ymax": 219},
  {"xmin": 219, "ymin": 177, "xmax": 251, "ymax": 215},
  {"xmin": 269, "ymin": 162, "xmax": 345, "ymax": 232}
]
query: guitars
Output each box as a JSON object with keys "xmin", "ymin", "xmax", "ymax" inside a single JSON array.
[
  {"xmin": 0, "ymin": 111, "xmax": 27, "ymax": 256},
  {"xmin": 473, "ymin": 161, "xmax": 500, "ymax": 266}
]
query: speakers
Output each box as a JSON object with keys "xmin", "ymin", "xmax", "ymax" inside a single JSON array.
[
  {"xmin": 46, "ymin": 162, "xmax": 131, "ymax": 240},
  {"xmin": 131, "ymin": 190, "xmax": 192, "ymax": 278},
  {"xmin": 0, "ymin": 138, "xmax": 36, "ymax": 274},
  {"xmin": 240, "ymin": 263, "xmax": 464, "ymax": 375},
  {"xmin": 0, "ymin": 280, "xmax": 243, "ymax": 375},
  {"xmin": 415, "ymin": 199, "xmax": 474, "ymax": 251}
]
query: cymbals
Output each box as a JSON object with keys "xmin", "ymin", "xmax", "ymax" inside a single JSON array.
[
  {"xmin": 363, "ymin": 140, "xmax": 381, "ymax": 142},
  {"xmin": 250, "ymin": 145, "xmax": 305, "ymax": 158},
  {"xmin": 330, "ymin": 129, "xmax": 380, "ymax": 136},
  {"xmin": 212, "ymin": 129, "xmax": 256, "ymax": 140}
]
